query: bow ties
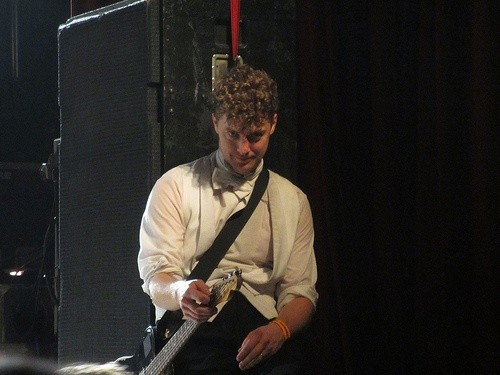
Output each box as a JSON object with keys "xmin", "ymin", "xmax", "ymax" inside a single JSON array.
[{"xmin": 212, "ymin": 167, "xmax": 253, "ymax": 199}]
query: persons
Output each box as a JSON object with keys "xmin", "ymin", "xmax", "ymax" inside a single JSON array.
[{"xmin": 136, "ymin": 63, "xmax": 321, "ymax": 375}]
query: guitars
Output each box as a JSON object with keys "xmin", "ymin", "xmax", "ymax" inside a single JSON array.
[{"xmin": 113, "ymin": 268, "xmax": 243, "ymax": 375}]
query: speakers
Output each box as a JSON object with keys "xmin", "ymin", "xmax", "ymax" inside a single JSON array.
[{"xmin": 58, "ymin": 0, "xmax": 297, "ymax": 375}]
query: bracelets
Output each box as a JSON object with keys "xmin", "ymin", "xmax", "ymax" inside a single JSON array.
[{"xmin": 269, "ymin": 319, "xmax": 292, "ymax": 341}]
